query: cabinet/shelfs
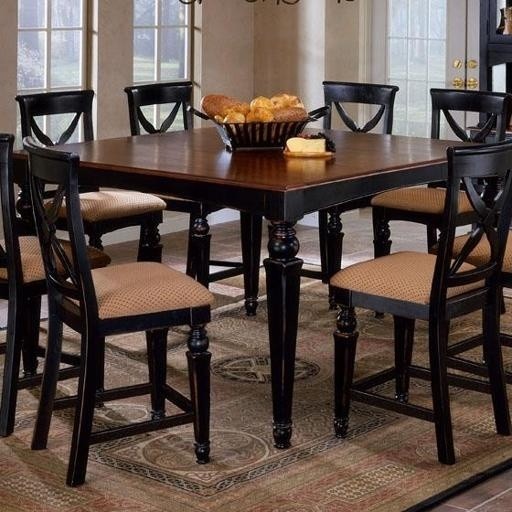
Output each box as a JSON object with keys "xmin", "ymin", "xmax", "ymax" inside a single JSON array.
[{"xmin": 466, "ymin": 0, "xmax": 512, "ymax": 230}]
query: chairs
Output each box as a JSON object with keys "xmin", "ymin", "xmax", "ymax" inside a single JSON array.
[
  {"xmin": 0, "ymin": 133, "xmax": 111, "ymax": 437},
  {"xmin": 371, "ymin": 89, "xmax": 512, "ymax": 319},
  {"xmin": 23, "ymin": 136, "xmax": 216, "ymax": 487},
  {"xmin": 432, "ymin": 227, "xmax": 512, "ymax": 384},
  {"xmin": 329, "ymin": 139, "xmax": 512, "ymax": 464},
  {"xmin": 125, "ymin": 82, "xmax": 262, "ymax": 317},
  {"xmin": 268, "ymin": 82, "xmax": 399, "ymax": 283},
  {"xmin": 15, "ymin": 90, "xmax": 167, "ymax": 263}
]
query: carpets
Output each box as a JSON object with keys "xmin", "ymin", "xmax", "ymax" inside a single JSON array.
[{"xmin": 0, "ymin": 262, "xmax": 512, "ymax": 512}]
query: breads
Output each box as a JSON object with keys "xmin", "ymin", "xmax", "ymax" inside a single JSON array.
[{"xmin": 200, "ymin": 92, "xmax": 309, "ymax": 142}]
